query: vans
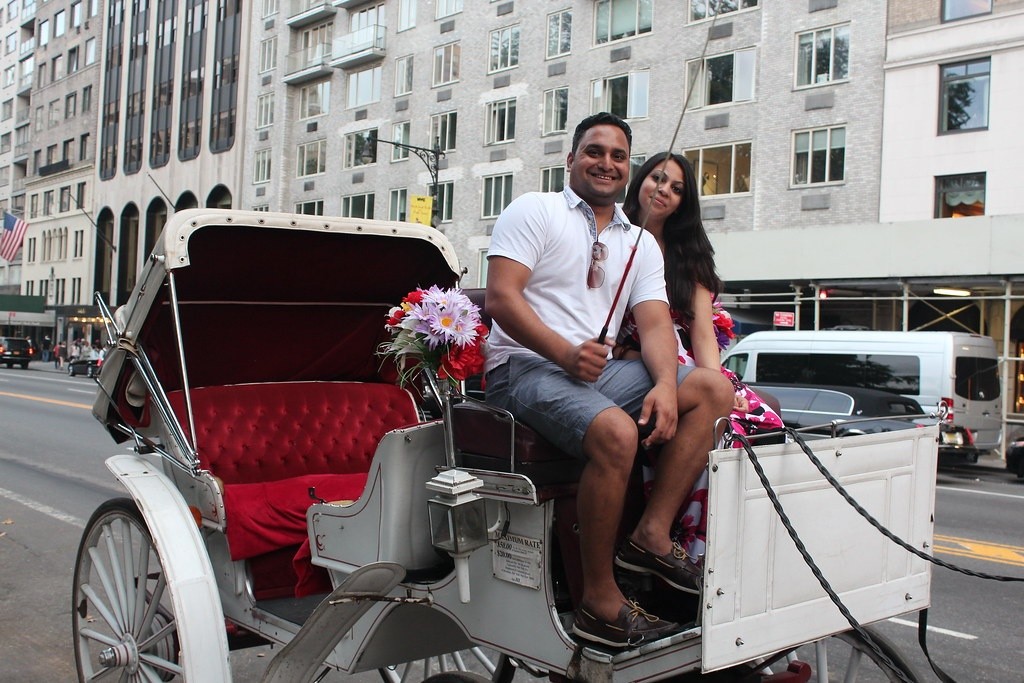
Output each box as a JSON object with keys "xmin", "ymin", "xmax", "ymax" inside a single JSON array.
[{"xmin": 720, "ymin": 329, "xmax": 1003, "ymax": 475}]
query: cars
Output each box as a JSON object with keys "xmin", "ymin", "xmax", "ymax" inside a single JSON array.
[{"xmin": 67, "ymin": 349, "xmax": 103, "ymax": 378}]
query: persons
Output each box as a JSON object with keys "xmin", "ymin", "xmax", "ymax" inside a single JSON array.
[
  {"xmin": 608, "ymin": 150, "xmax": 785, "ymax": 571},
  {"xmin": 483, "ymin": 111, "xmax": 737, "ymax": 647},
  {"xmin": 25, "ymin": 335, "xmax": 111, "ymax": 370}
]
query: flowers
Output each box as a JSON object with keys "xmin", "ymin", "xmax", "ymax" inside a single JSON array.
[
  {"xmin": 374, "ymin": 285, "xmax": 492, "ymax": 404},
  {"xmin": 711, "ymin": 298, "xmax": 737, "ymax": 353}
]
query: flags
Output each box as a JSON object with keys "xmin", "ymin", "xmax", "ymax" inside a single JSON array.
[{"xmin": 0, "ymin": 210, "xmax": 29, "ymax": 263}]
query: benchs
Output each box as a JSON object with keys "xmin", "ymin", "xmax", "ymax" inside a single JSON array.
[
  {"xmin": 159, "ymin": 384, "xmax": 427, "ymax": 603},
  {"xmin": 448, "ymin": 285, "xmax": 781, "ymax": 630}
]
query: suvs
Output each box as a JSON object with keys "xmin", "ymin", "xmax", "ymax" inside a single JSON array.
[{"xmin": 0, "ymin": 335, "xmax": 34, "ymax": 369}]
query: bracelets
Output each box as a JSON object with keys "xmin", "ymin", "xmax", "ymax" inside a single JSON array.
[{"xmin": 616, "ymin": 344, "xmax": 635, "ymax": 360}]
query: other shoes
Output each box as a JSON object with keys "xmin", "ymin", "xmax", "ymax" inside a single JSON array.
[
  {"xmin": 572, "ymin": 598, "xmax": 680, "ymax": 648},
  {"xmin": 615, "ymin": 538, "xmax": 706, "ymax": 595}
]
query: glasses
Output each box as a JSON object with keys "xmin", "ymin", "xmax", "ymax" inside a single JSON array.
[{"xmin": 587, "ymin": 242, "xmax": 609, "ymax": 289}]
